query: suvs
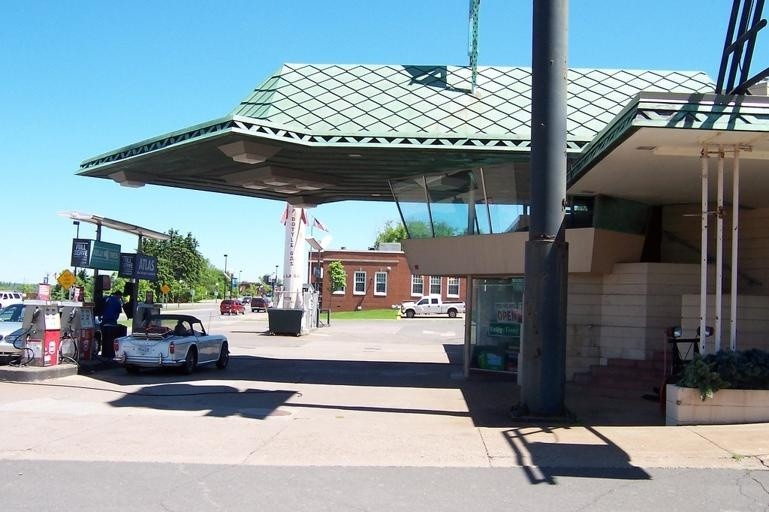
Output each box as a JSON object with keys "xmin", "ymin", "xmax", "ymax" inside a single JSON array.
[
  {"xmin": 251, "ymin": 297, "xmax": 269, "ymax": 313},
  {"xmin": 219, "ymin": 300, "xmax": 246, "ymax": 315}
]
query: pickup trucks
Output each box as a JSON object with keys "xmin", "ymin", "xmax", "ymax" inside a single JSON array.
[{"xmin": 400, "ymin": 295, "xmax": 465, "ymax": 318}]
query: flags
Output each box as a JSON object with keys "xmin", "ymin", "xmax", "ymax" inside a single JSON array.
[
  {"xmin": 300, "ymin": 208, "xmax": 309, "ymax": 226},
  {"xmin": 279, "ymin": 207, "xmax": 288, "ymax": 226},
  {"xmin": 312, "ymin": 217, "xmax": 330, "ymax": 233}
]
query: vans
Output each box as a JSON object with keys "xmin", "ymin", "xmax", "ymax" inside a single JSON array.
[
  {"xmin": 0, "ymin": 301, "xmax": 102, "ymax": 363},
  {"xmin": 0, "ymin": 291, "xmax": 23, "ymax": 309}
]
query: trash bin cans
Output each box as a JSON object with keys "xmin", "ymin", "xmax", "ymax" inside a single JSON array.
[{"xmin": 267, "ymin": 309, "xmax": 303, "ymax": 337}]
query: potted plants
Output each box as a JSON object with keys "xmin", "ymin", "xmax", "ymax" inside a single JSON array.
[{"xmin": 665, "ymin": 348, "xmax": 769, "ymax": 426}]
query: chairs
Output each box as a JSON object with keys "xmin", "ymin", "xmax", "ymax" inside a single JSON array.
[{"xmin": 175, "ymin": 323, "xmax": 186, "ymax": 336}]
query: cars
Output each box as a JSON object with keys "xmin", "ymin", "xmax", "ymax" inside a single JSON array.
[
  {"xmin": 114, "ymin": 314, "xmax": 229, "ymax": 376},
  {"xmin": 243, "ymin": 296, "xmax": 252, "ymax": 303}
]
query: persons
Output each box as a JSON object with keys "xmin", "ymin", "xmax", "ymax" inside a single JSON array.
[{"xmin": 99, "ymin": 290, "xmax": 123, "ymax": 329}]
